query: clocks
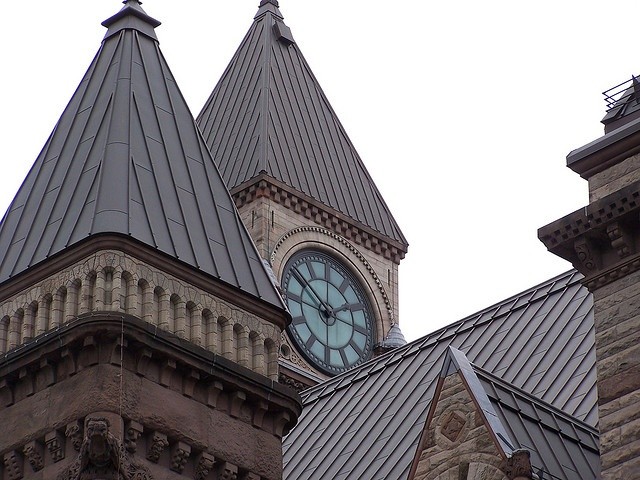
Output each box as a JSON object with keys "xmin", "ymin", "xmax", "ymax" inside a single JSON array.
[{"xmin": 281, "ymin": 249, "xmax": 378, "ymax": 378}]
